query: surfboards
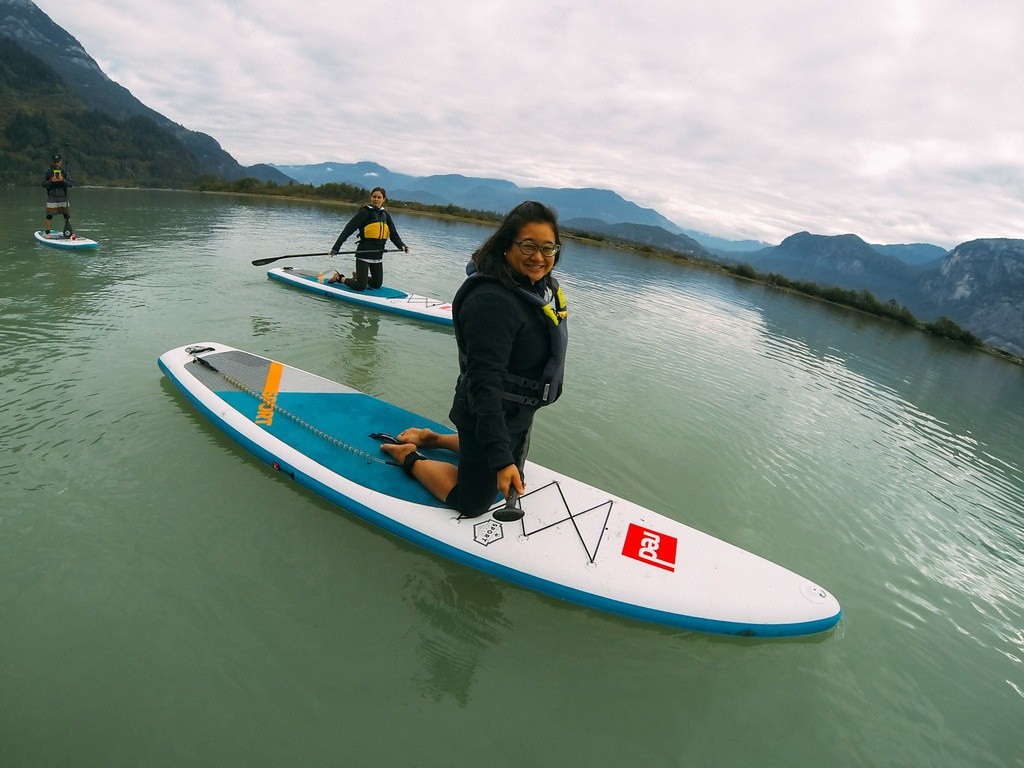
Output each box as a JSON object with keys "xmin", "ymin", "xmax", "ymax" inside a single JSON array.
[
  {"xmin": 33, "ymin": 229, "xmax": 98, "ymax": 250},
  {"xmin": 267, "ymin": 266, "xmax": 454, "ymax": 327},
  {"xmin": 157, "ymin": 341, "xmax": 842, "ymax": 637}
]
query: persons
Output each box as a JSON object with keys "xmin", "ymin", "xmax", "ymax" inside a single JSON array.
[
  {"xmin": 328, "ymin": 187, "xmax": 409, "ymax": 291},
  {"xmin": 381, "ymin": 200, "xmax": 568, "ymax": 519},
  {"xmin": 41, "ymin": 154, "xmax": 73, "ymax": 238}
]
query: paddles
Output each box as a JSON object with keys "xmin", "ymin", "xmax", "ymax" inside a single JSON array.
[
  {"xmin": 492, "ymin": 416, "xmax": 534, "ymax": 521},
  {"xmin": 63, "ymin": 160, "xmax": 73, "ymax": 238},
  {"xmin": 252, "ymin": 248, "xmax": 407, "ymax": 266}
]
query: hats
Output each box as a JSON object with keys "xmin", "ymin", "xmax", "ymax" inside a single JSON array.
[{"xmin": 52, "ymin": 154, "xmax": 61, "ymax": 161}]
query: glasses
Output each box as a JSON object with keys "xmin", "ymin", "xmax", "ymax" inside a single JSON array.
[{"xmin": 513, "ymin": 238, "xmax": 562, "ymax": 258}]
